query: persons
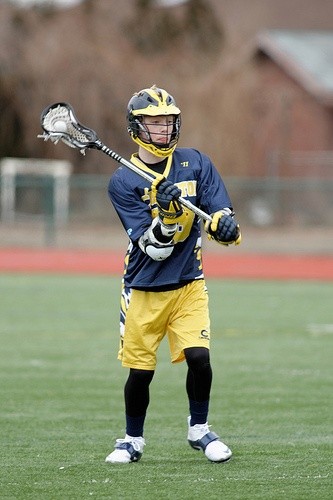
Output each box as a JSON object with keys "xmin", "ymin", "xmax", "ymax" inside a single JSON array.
[{"xmin": 105, "ymin": 84, "xmax": 242, "ymax": 465}]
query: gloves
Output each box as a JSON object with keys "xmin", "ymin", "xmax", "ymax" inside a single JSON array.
[
  {"xmin": 212, "ymin": 212, "xmax": 241, "ymax": 246},
  {"xmin": 156, "ymin": 181, "xmax": 186, "ymax": 224}
]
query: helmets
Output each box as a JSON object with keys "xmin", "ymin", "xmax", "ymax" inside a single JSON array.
[{"xmin": 127, "ymin": 88, "xmax": 181, "ymax": 156}]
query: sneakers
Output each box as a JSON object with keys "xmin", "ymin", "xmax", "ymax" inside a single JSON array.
[
  {"xmin": 187, "ymin": 416, "xmax": 231, "ymax": 462},
  {"xmin": 105, "ymin": 438, "xmax": 145, "ymax": 463}
]
